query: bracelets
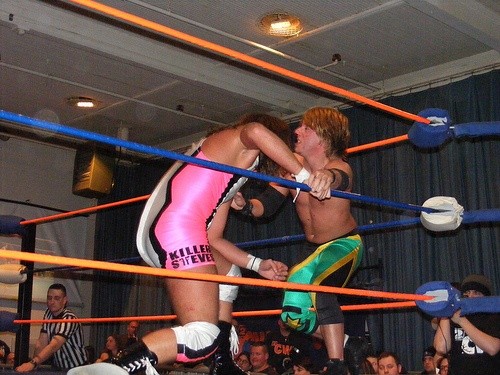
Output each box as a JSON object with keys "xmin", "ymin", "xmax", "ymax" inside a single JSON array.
[
  {"xmin": 292, "ymin": 167, "xmax": 310, "ymax": 183},
  {"xmin": 239, "ymin": 200, "xmax": 252, "ymax": 215},
  {"xmin": 246, "ymin": 254, "xmax": 263, "ymax": 273},
  {"xmin": 328, "ymin": 169, "xmax": 337, "ymax": 185}
]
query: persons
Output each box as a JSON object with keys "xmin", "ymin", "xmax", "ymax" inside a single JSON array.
[
  {"xmin": 15, "ymin": 284, "xmax": 88, "ymax": 372},
  {"xmin": 66, "ymin": 113, "xmax": 331, "ymax": 374},
  {"xmin": 0, "ymin": 306, "xmax": 448, "ymax": 375},
  {"xmin": 230, "ymin": 107, "xmax": 364, "ymax": 375},
  {"xmin": 432, "ymin": 275, "xmax": 500, "ymax": 375}
]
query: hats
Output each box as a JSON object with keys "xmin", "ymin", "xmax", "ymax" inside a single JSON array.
[{"xmin": 461, "ymin": 274, "xmax": 493, "ymax": 293}]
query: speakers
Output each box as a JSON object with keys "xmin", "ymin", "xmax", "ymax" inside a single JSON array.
[{"xmin": 72, "ymin": 145, "xmax": 116, "ymax": 198}]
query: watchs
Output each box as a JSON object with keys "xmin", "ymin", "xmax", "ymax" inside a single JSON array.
[{"xmin": 30, "ymin": 359, "xmax": 38, "ymax": 367}]
